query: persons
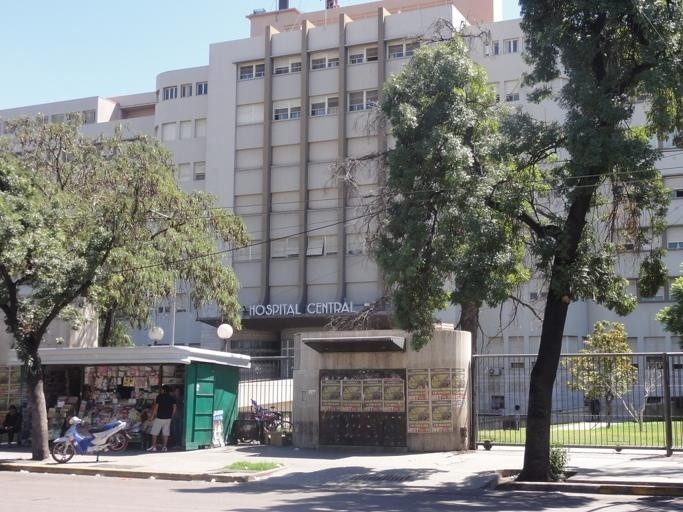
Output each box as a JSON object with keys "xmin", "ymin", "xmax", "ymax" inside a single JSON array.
[
  {"xmin": 0, "ymin": 405, "xmax": 21, "ymax": 447},
  {"xmin": 145, "ymin": 385, "xmax": 176, "ymax": 451}
]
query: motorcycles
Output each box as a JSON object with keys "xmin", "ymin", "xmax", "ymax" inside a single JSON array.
[{"xmin": 50, "ymin": 412, "xmax": 133, "ymax": 466}]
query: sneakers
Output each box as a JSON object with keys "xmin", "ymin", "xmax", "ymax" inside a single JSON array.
[
  {"xmin": 160, "ymin": 447, "xmax": 167, "ymax": 452},
  {"xmin": 147, "ymin": 446, "xmax": 156, "ymax": 452}
]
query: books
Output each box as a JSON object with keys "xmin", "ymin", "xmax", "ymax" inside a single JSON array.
[{"xmin": 47, "ymin": 366, "xmax": 160, "ymax": 439}]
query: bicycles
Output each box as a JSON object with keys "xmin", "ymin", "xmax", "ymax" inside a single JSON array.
[{"xmin": 234, "ymin": 398, "xmax": 294, "ymax": 452}]
